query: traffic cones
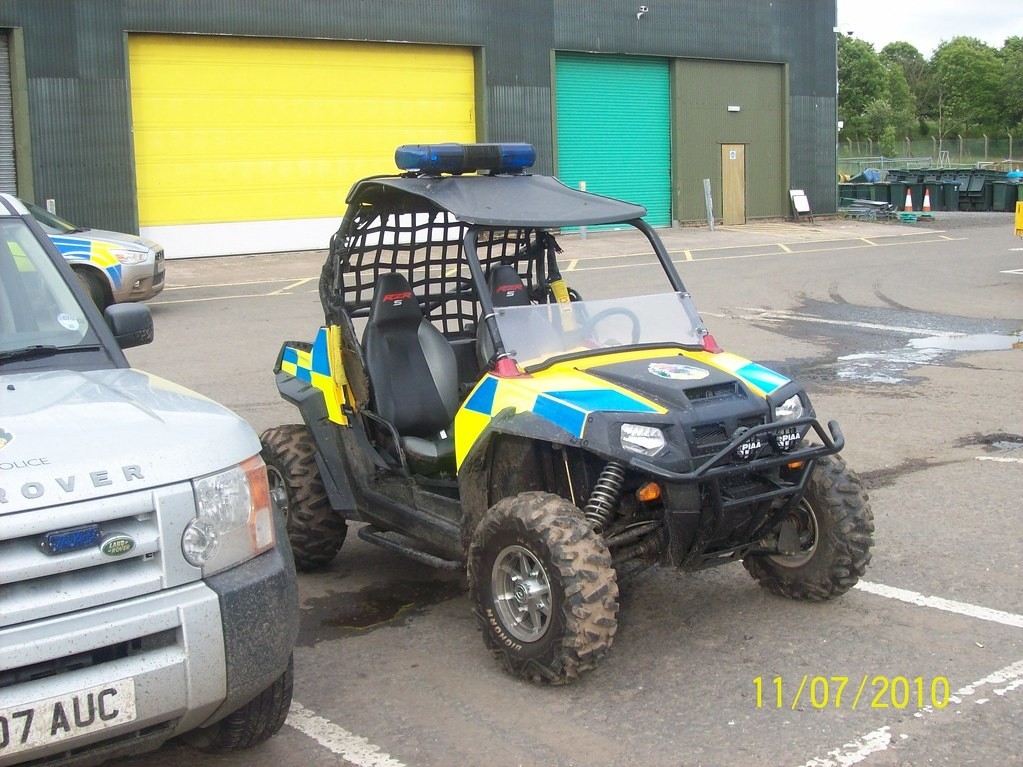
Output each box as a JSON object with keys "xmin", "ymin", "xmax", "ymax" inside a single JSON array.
[
  {"xmin": 904, "ymin": 188, "xmax": 913, "ymax": 211},
  {"xmin": 922, "ymin": 188, "xmax": 932, "ymax": 213}
]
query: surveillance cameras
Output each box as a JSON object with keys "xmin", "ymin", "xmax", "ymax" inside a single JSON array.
[
  {"xmin": 639, "ymin": 6, "xmax": 649, "ymax": 12},
  {"xmin": 838, "ymin": 23, "xmax": 854, "ymax": 36}
]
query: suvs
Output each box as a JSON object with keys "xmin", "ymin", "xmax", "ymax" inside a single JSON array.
[{"xmin": 258, "ymin": 145, "xmax": 874, "ymax": 689}]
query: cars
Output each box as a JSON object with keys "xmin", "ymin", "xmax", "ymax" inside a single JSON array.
[
  {"xmin": 27, "ymin": 199, "xmax": 167, "ymax": 303},
  {"xmin": 2, "ymin": 192, "xmax": 297, "ymax": 749}
]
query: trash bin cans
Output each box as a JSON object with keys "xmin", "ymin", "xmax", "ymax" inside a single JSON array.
[{"xmin": 838, "ymin": 166, "xmax": 1023, "ymax": 213}]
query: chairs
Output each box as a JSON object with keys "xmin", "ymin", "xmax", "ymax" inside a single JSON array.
[
  {"xmin": 361, "ymin": 273, "xmax": 460, "ymax": 479},
  {"xmin": 475, "ymin": 265, "xmax": 562, "ymax": 370}
]
